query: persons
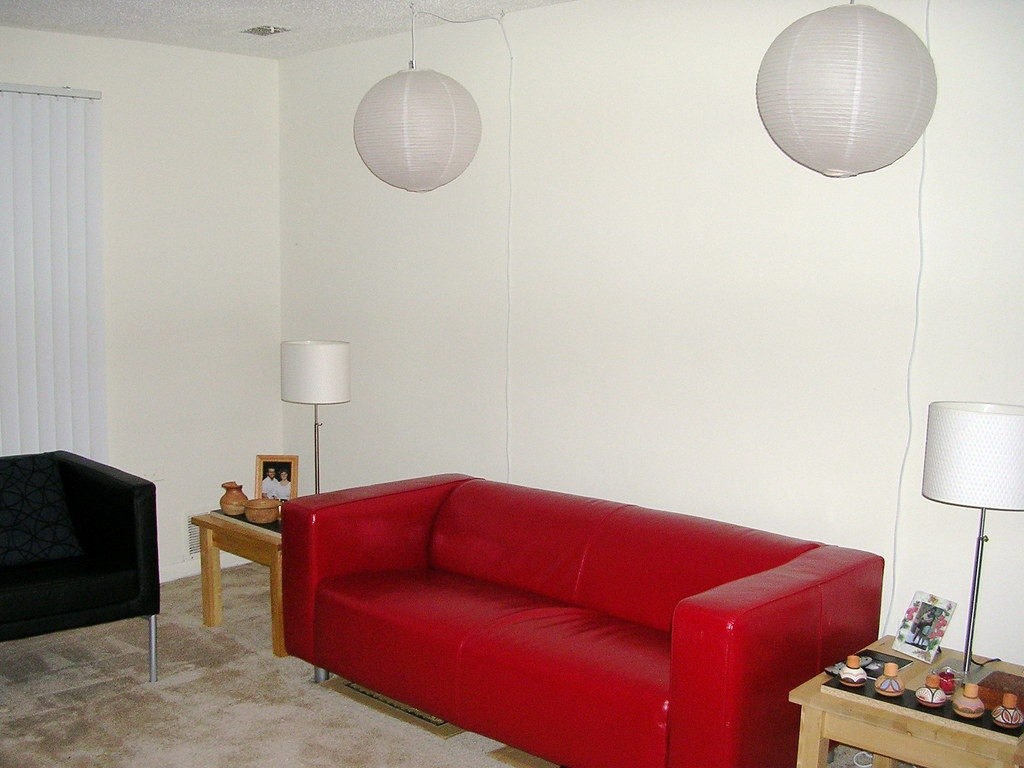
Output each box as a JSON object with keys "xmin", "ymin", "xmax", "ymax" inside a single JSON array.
[
  {"xmin": 910, "ymin": 606, "xmax": 937, "ymax": 649},
  {"xmin": 262, "ymin": 468, "xmax": 292, "ymax": 501}
]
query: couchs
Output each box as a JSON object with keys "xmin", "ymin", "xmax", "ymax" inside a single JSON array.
[
  {"xmin": 0, "ymin": 451, "xmax": 160, "ymax": 682},
  {"xmin": 280, "ymin": 473, "xmax": 885, "ymax": 768}
]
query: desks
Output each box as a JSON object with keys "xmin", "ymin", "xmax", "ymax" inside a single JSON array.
[
  {"xmin": 190, "ymin": 515, "xmax": 288, "ymax": 657},
  {"xmin": 788, "ymin": 635, "xmax": 1024, "ymax": 768}
]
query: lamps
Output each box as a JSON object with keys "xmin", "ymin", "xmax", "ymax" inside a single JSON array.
[
  {"xmin": 921, "ymin": 401, "xmax": 1024, "ymax": 688},
  {"xmin": 281, "ymin": 339, "xmax": 351, "ymax": 494}
]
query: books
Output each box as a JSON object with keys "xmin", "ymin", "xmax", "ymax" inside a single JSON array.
[{"xmin": 823, "ymin": 649, "xmax": 913, "ymax": 681}]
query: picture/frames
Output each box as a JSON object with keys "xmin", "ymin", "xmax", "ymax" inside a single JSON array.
[
  {"xmin": 893, "ymin": 590, "xmax": 957, "ymax": 663},
  {"xmin": 255, "ymin": 455, "xmax": 298, "ymax": 500}
]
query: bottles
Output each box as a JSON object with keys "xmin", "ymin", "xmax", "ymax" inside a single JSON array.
[
  {"xmin": 914, "ymin": 675, "xmax": 946, "ymax": 706},
  {"xmin": 952, "ymin": 683, "xmax": 984, "ymax": 717},
  {"xmin": 219, "ymin": 481, "xmax": 248, "ymax": 516},
  {"xmin": 990, "ymin": 693, "xmax": 1024, "ymax": 729},
  {"xmin": 874, "ymin": 662, "xmax": 905, "ymax": 698},
  {"xmin": 837, "ymin": 656, "xmax": 867, "ymax": 687}
]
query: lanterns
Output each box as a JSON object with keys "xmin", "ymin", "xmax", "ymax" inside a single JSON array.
[
  {"xmin": 756, "ymin": 5, "xmax": 936, "ymax": 177},
  {"xmin": 354, "ymin": 67, "xmax": 481, "ymax": 192}
]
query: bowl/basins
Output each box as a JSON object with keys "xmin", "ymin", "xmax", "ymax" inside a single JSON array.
[{"xmin": 242, "ymin": 498, "xmax": 283, "ymax": 523}]
